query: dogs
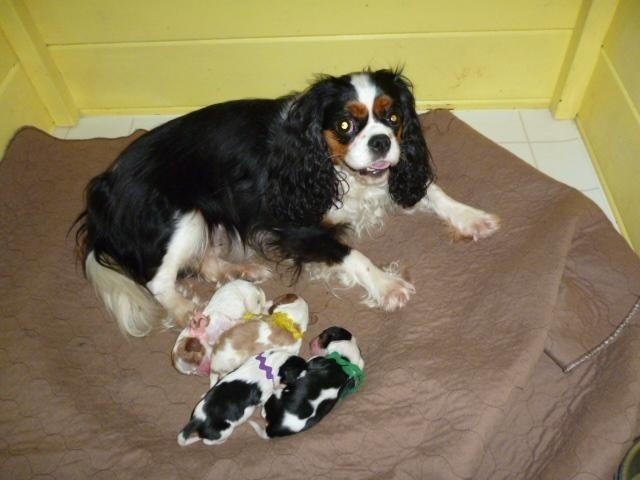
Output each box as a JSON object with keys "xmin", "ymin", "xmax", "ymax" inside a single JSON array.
[
  {"xmin": 170, "ymin": 277, "xmax": 365, "ymax": 447},
  {"xmin": 67, "ymin": 63, "xmax": 501, "ymax": 330}
]
